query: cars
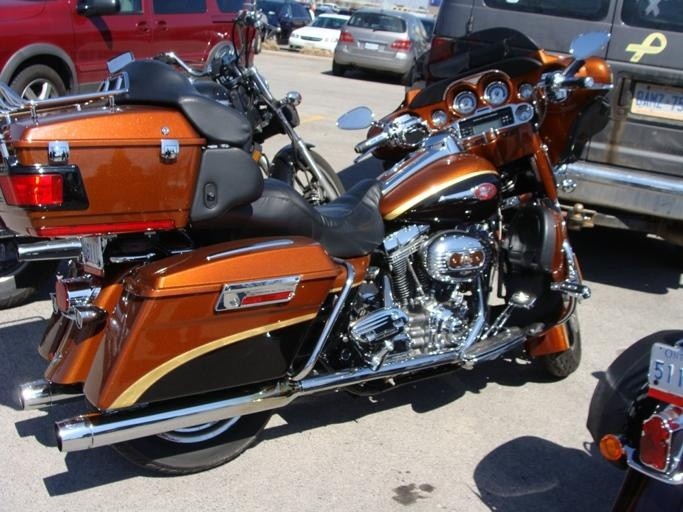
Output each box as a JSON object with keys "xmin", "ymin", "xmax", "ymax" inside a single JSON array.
[
  {"xmin": 0, "ymin": 0, "xmax": 256, "ymax": 104},
  {"xmin": 288, "ymin": 13, "xmax": 350, "ymax": 55},
  {"xmin": 332, "ymin": 9, "xmax": 430, "ymax": 85},
  {"xmin": 257, "ymin": 0, "xmax": 312, "ymax": 44}
]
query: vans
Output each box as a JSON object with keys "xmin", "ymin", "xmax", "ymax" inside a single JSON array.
[{"xmin": 427, "ymin": 0, "xmax": 683, "ymax": 249}]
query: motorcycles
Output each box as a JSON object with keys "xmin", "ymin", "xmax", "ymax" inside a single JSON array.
[
  {"xmin": 0, "ymin": 26, "xmax": 611, "ymax": 477},
  {"xmin": 587, "ymin": 329, "xmax": 683, "ymax": 512},
  {"xmin": 0, "ymin": 13, "xmax": 346, "ymax": 308}
]
